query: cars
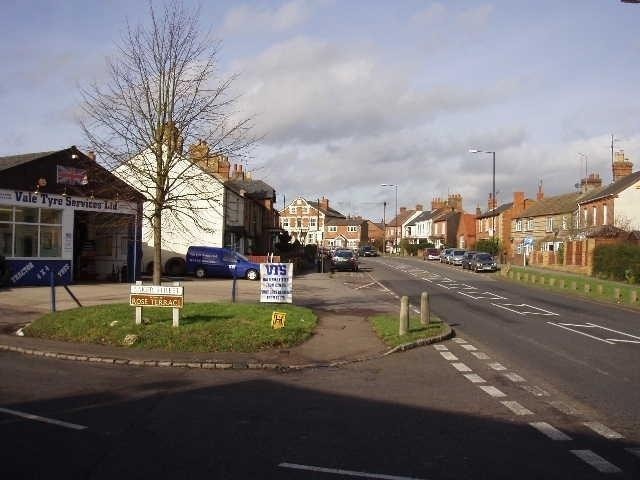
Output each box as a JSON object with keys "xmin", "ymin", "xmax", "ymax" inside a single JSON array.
[
  {"xmin": 320, "ymin": 245, "xmax": 377, "ymax": 273},
  {"xmin": 422, "ymin": 248, "xmax": 497, "ymax": 273}
]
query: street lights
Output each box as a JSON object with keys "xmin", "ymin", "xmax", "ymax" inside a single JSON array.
[
  {"xmin": 382, "ymin": 202, "xmax": 387, "ymax": 256},
  {"xmin": 470, "ymin": 148, "xmax": 495, "ymax": 265},
  {"xmin": 380, "ymin": 183, "xmax": 398, "ymax": 256},
  {"xmin": 579, "ymin": 151, "xmax": 588, "ymax": 192}
]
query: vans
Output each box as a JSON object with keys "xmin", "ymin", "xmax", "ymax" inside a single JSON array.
[{"xmin": 186, "ymin": 246, "xmax": 260, "ymax": 281}]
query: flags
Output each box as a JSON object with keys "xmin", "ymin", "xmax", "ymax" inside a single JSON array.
[{"xmin": 56, "ymin": 165, "xmax": 88, "ymax": 186}]
